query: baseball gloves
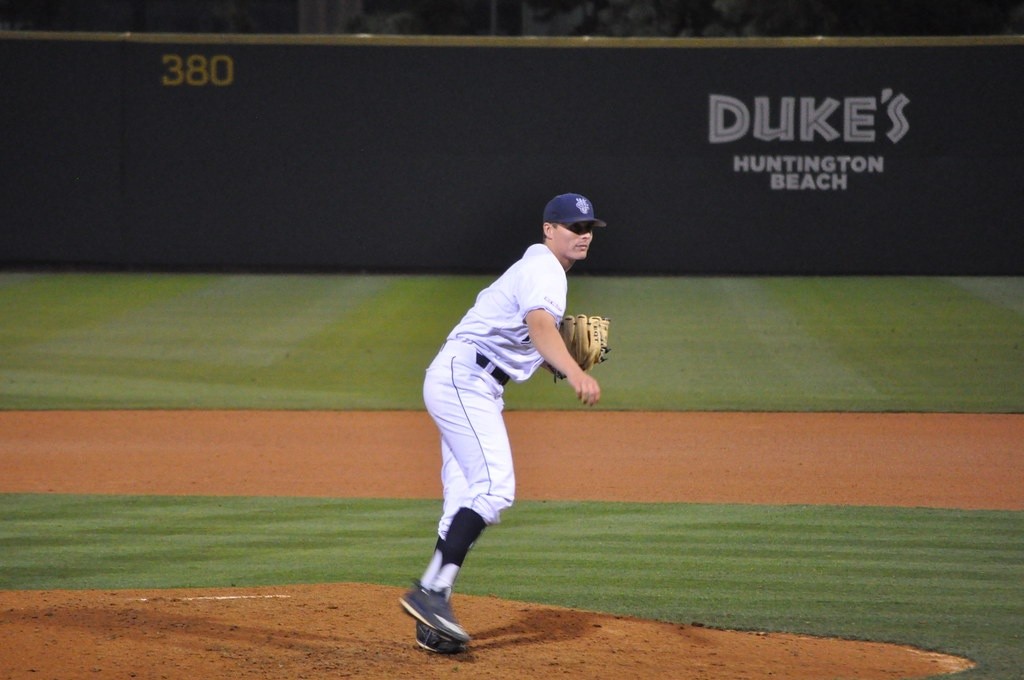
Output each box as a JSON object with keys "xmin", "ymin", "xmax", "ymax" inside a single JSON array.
[{"xmin": 549, "ymin": 314, "xmax": 611, "ymax": 379}]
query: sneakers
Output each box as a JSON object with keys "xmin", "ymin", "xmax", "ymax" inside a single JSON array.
[
  {"xmin": 414, "ymin": 619, "xmax": 466, "ymax": 655},
  {"xmin": 399, "ymin": 584, "xmax": 471, "ymax": 642}
]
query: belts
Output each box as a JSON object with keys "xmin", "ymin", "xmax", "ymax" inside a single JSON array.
[{"xmin": 476, "ymin": 352, "xmax": 510, "ymax": 387}]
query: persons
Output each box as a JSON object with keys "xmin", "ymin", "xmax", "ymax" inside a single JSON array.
[{"xmin": 400, "ymin": 193, "xmax": 610, "ymax": 654}]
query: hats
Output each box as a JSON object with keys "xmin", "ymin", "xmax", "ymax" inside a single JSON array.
[{"xmin": 543, "ymin": 192, "xmax": 606, "ymax": 228}]
